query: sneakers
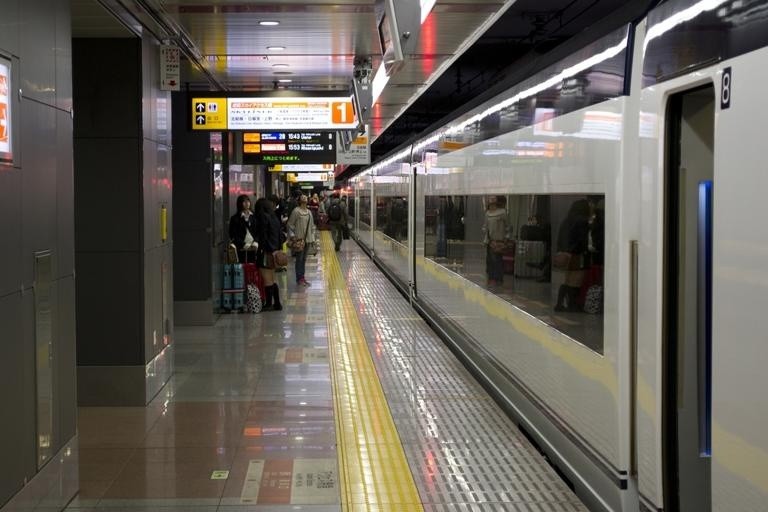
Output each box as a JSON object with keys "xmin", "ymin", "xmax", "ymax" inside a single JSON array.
[{"xmin": 298, "ymin": 276, "xmax": 310, "ymax": 285}]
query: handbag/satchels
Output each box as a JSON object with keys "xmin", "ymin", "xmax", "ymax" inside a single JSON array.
[
  {"xmin": 269, "ymin": 250, "xmax": 289, "ymax": 268},
  {"xmin": 489, "ymin": 240, "xmax": 508, "ymax": 254},
  {"xmin": 521, "ymin": 216, "xmax": 547, "ymax": 241},
  {"xmin": 286, "ymin": 239, "xmax": 305, "ymax": 252},
  {"xmin": 552, "ymin": 251, "xmax": 577, "ymax": 270},
  {"xmin": 343, "ymin": 223, "xmax": 350, "ymax": 239}
]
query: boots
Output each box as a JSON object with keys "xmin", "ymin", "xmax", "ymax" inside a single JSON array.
[
  {"xmin": 262, "ymin": 283, "xmax": 282, "ymax": 310},
  {"xmin": 554, "ymin": 284, "xmax": 582, "ymax": 312}
]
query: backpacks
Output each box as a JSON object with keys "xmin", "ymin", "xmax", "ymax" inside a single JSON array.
[
  {"xmin": 225, "ymin": 242, "xmax": 239, "ymax": 263},
  {"xmin": 583, "ymin": 285, "xmax": 602, "ymax": 314},
  {"xmin": 328, "ymin": 199, "xmax": 342, "ymax": 221}
]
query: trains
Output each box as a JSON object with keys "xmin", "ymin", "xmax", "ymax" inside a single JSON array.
[{"xmin": 340, "ymin": 0, "xmax": 768, "ymax": 512}]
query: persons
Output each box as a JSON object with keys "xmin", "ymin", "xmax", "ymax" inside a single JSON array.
[
  {"xmin": 524, "ymin": 249, "xmax": 552, "ymax": 282},
  {"xmin": 482, "ymin": 196, "xmax": 510, "ymax": 287},
  {"xmin": 432, "ymin": 196, "xmax": 466, "ymax": 260},
  {"xmin": 553, "ymin": 195, "xmax": 604, "ymax": 314},
  {"xmin": 377, "ymin": 197, "xmax": 408, "ymax": 243},
  {"xmin": 228, "ymin": 187, "xmax": 348, "ymax": 310}
]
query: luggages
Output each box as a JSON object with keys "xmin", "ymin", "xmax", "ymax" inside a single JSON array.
[
  {"xmin": 222, "ymin": 246, "xmax": 266, "ymax": 313},
  {"xmin": 514, "ymin": 240, "xmax": 547, "ymax": 279}
]
